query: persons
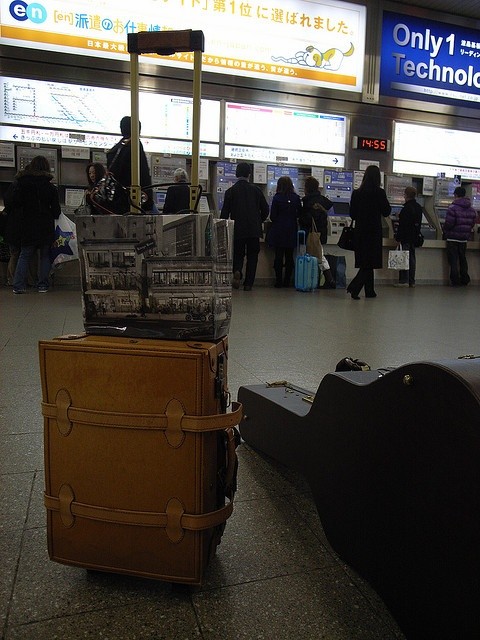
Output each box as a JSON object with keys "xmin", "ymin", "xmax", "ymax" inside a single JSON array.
[
  {"xmin": 299, "ymin": 176, "xmax": 337, "ymax": 289},
  {"xmin": 346, "ymin": 165, "xmax": 392, "ymax": 300},
  {"xmin": 162, "ymin": 168, "xmax": 190, "ymax": 215},
  {"xmin": 1, "ymin": 156, "xmax": 62, "ymax": 294},
  {"xmin": 393, "ymin": 186, "xmax": 422, "ymax": 288},
  {"xmin": 1, "ymin": 165, "xmax": 36, "ymax": 288},
  {"xmin": 219, "ymin": 163, "xmax": 269, "ymax": 291},
  {"xmin": 443, "ymin": 187, "xmax": 476, "ymax": 286},
  {"xmin": 85, "ymin": 163, "xmax": 114, "ymax": 217},
  {"xmin": 270, "ymin": 176, "xmax": 303, "ymax": 288},
  {"xmin": 106, "ymin": 116, "xmax": 160, "ymax": 215}
]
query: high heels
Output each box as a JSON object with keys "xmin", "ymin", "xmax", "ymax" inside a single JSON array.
[
  {"xmin": 346, "ymin": 288, "xmax": 360, "ymax": 300},
  {"xmin": 365, "ymin": 291, "xmax": 378, "ymax": 298}
]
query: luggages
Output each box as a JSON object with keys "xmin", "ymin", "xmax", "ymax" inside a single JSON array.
[
  {"xmin": 295, "ymin": 230, "xmax": 320, "ymax": 293},
  {"xmin": 38, "ymin": 29, "xmax": 246, "ymax": 598}
]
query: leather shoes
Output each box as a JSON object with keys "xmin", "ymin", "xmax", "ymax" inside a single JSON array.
[
  {"xmin": 243, "ymin": 284, "xmax": 251, "ymax": 291},
  {"xmin": 232, "ymin": 270, "xmax": 241, "ymax": 290}
]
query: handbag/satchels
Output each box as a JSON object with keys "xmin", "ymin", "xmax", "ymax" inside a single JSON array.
[
  {"xmin": 80, "ymin": 180, "xmax": 235, "ymax": 342},
  {"xmin": 89, "ymin": 171, "xmax": 129, "ymax": 216},
  {"xmin": 322, "ymin": 253, "xmax": 347, "ymax": 290},
  {"xmin": 306, "ymin": 214, "xmax": 323, "ymax": 263},
  {"xmin": 387, "ymin": 241, "xmax": 409, "ymax": 270},
  {"xmin": 407, "ymin": 201, "xmax": 424, "ymax": 246},
  {"xmin": 337, "ymin": 218, "xmax": 356, "ymax": 251},
  {"xmin": 75, "ymin": 190, "xmax": 91, "ymax": 215},
  {"xmin": 53, "ymin": 209, "xmax": 79, "ymax": 266}
]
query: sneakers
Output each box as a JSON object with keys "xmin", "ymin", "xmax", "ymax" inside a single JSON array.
[
  {"xmin": 38, "ymin": 286, "xmax": 49, "ymax": 293},
  {"xmin": 12, "ymin": 289, "xmax": 35, "ymax": 294}
]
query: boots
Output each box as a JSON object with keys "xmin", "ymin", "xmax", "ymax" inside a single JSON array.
[
  {"xmin": 283, "ymin": 257, "xmax": 293, "ymax": 288},
  {"xmin": 317, "ymin": 269, "xmax": 337, "ymax": 289},
  {"xmin": 273, "ymin": 257, "xmax": 283, "ymax": 288}
]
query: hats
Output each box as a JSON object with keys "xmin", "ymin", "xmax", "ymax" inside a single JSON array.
[
  {"xmin": 453, "ymin": 187, "xmax": 466, "ymax": 195},
  {"xmin": 405, "ymin": 187, "xmax": 417, "ymax": 195},
  {"xmin": 120, "ymin": 115, "xmax": 141, "ymax": 137}
]
